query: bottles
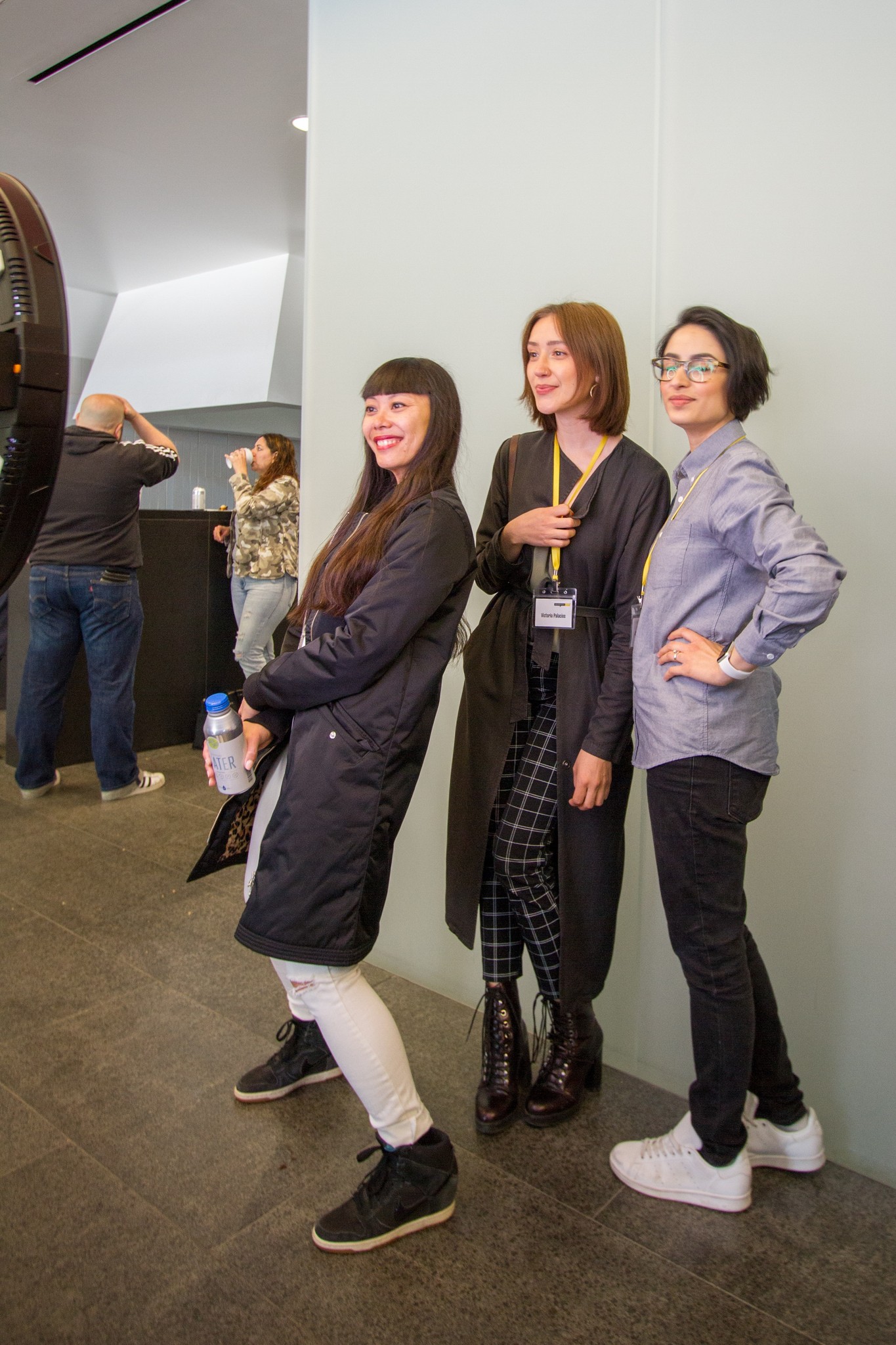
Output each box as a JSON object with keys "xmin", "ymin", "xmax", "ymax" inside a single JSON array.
[{"xmin": 203, "ymin": 693, "xmax": 256, "ymax": 795}]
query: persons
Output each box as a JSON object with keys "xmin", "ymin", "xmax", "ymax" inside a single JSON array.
[
  {"xmin": 14, "ymin": 393, "xmax": 180, "ymax": 800},
  {"xmin": 607, "ymin": 308, "xmax": 847, "ymax": 1213},
  {"xmin": 186, "ymin": 356, "xmax": 478, "ymax": 1251},
  {"xmin": 213, "ymin": 433, "xmax": 300, "ymax": 681},
  {"xmin": 444, "ymin": 302, "xmax": 672, "ymax": 1142}
]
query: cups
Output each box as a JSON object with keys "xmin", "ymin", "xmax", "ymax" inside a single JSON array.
[{"xmin": 226, "ymin": 447, "xmax": 253, "ymax": 468}]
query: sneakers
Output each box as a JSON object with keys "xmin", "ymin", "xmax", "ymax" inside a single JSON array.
[
  {"xmin": 21, "ymin": 770, "xmax": 60, "ymax": 799},
  {"xmin": 742, "ymin": 1089, "xmax": 826, "ymax": 1172},
  {"xmin": 609, "ymin": 1111, "xmax": 752, "ymax": 1212},
  {"xmin": 119, "ymin": 769, "xmax": 164, "ymax": 798}
]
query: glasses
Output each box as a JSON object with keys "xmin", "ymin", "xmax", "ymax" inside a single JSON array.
[{"xmin": 652, "ymin": 358, "xmax": 731, "ymax": 383}]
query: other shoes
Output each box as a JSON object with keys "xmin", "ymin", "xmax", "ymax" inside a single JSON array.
[
  {"xmin": 234, "ymin": 1014, "xmax": 342, "ymax": 1102},
  {"xmin": 311, "ymin": 1129, "xmax": 457, "ymax": 1251}
]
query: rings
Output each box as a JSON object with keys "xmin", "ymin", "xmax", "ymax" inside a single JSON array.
[{"xmin": 673, "ymin": 649, "xmax": 680, "ymax": 663}]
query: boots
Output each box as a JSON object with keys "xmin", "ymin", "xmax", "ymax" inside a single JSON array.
[
  {"xmin": 526, "ymin": 1006, "xmax": 603, "ymax": 1126},
  {"xmin": 475, "ymin": 979, "xmax": 532, "ymax": 1133}
]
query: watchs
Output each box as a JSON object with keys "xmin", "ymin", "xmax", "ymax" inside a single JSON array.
[{"xmin": 717, "ymin": 641, "xmax": 755, "ymax": 680}]
